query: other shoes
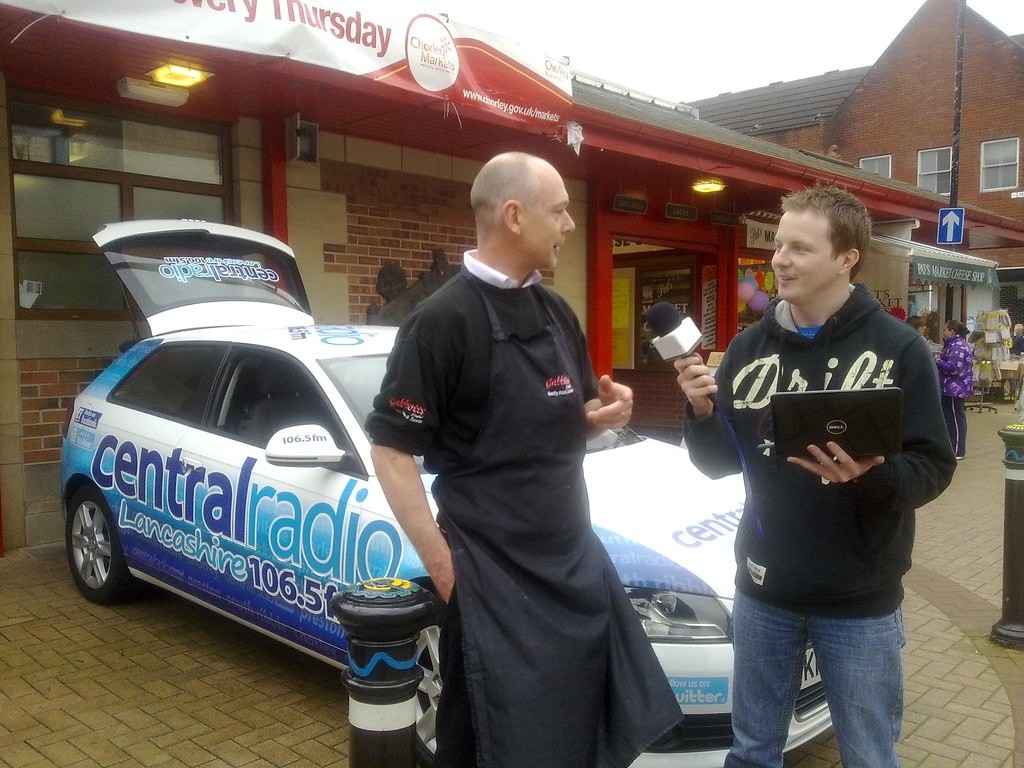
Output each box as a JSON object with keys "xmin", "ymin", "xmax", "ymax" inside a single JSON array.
[{"xmin": 956, "ymin": 454, "xmax": 966, "ymax": 460}]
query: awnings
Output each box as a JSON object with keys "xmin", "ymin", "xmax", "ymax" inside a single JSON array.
[{"xmin": 869, "ymin": 232, "xmax": 999, "ymax": 291}]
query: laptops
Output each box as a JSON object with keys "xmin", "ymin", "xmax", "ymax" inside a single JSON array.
[{"xmin": 767, "ymin": 387, "xmax": 905, "ymax": 459}]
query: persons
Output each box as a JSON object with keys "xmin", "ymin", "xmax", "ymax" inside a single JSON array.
[
  {"xmin": 676, "ymin": 186, "xmax": 956, "ymax": 768},
  {"xmin": 934, "ymin": 320, "xmax": 974, "ymax": 459},
  {"xmin": 1009, "ymin": 324, "xmax": 1024, "ymax": 390},
  {"xmin": 905, "ymin": 315, "xmax": 932, "ymax": 349},
  {"xmin": 364, "ymin": 152, "xmax": 685, "ymax": 768}
]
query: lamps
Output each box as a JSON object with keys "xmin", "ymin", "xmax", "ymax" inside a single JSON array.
[
  {"xmin": 690, "ymin": 177, "xmax": 728, "ymax": 193},
  {"xmin": 145, "ymin": 62, "xmax": 215, "ymax": 87}
]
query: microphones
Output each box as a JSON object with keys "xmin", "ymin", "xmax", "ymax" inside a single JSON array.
[{"xmin": 648, "ymin": 301, "xmax": 720, "ymax": 404}]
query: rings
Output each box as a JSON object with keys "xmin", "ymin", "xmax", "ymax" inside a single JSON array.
[{"xmin": 621, "ymin": 412, "xmax": 625, "ymax": 422}]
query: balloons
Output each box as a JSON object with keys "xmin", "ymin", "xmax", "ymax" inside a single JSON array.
[
  {"xmin": 738, "ymin": 282, "xmax": 754, "ymax": 302},
  {"xmin": 741, "ymin": 277, "xmax": 758, "ymax": 291},
  {"xmin": 738, "ymin": 300, "xmax": 746, "ymax": 313},
  {"xmin": 748, "ymin": 292, "xmax": 769, "ymax": 311}
]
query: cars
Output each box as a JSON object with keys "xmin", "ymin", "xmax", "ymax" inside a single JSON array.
[{"xmin": 57, "ymin": 216, "xmax": 832, "ymax": 767}]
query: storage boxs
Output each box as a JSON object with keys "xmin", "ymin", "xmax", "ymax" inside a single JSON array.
[{"xmin": 999, "ymin": 361, "xmax": 1020, "ymax": 379}]
